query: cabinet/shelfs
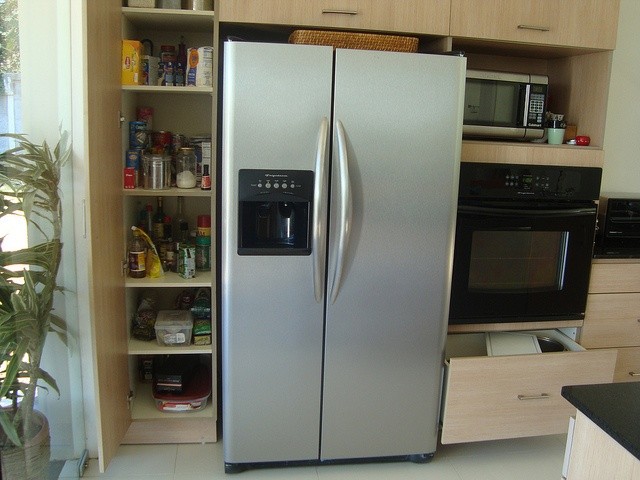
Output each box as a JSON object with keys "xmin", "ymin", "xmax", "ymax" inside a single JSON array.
[
  {"xmin": 450, "ymin": 35, "xmax": 614, "ymax": 149},
  {"xmin": 582, "ymin": 259, "xmax": 640, "ymax": 382},
  {"xmin": 219, "ymin": 0, "xmax": 619, "ymax": 50},
  {"xmin": 441, "ymin": 323, "xmax": 616, "ymax": 444},
  {"xmin": 120, "ymin": 0, "xmax": 217, "ymax": 444}
]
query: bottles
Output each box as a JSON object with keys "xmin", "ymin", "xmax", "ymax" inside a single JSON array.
[
  {"xmin": 143, "ymin": 155, "xmax": 172, "ymax": 190},
  {"xmin": 154, "ymin": 196, "xmax": 166, "ymax": 239},
  {"xmin": 159, "ymin": 45, "xmax": 178, "ymax": 65},
  {"xmin": 129, "ymin": 120, "xmax": 148, "ymax": 150},
  {"xmin": 176, "ymin": 223, "xmax": 190, "ymax": 247},
  {"xmin": 173, "ymin": 196, "xmax": 188, "ymax": 241},
  {"xmin": 176, "ymin": 45, "xmax": 187, "ymax": 66},
  {"xmin": 200, "ymin": 164, "xmax": 211, "ymax": 190},
  {"xmin": 175, "ymin": 147, "xmax": 197, "ymax": 189},
  {"xmin": 194, "ymin": 237, "xmax": 211, "ymax": 271},
  {"xmin": 159, "ymin": 216, "xmax": 178, "ymax": 272},
  {"xmin": 144, "ymin": 204, "xmax": 154, "ymax": 239},
  {"xmin": 129, "ymin": 230, "xmax": 147, "ymax": 279},
  {"xmin": 138, "ymin": 106, "xmax": 154, "ymax": 132}
]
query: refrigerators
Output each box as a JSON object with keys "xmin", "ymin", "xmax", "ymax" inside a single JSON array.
[{"xmin": 222, "ymin": 40, "xmax": 467, "ymax": 474}]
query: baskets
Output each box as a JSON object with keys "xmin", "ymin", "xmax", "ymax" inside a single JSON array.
[{"xmin": 287, "ymin": 29, "xmax": 419, "ymax": 53}]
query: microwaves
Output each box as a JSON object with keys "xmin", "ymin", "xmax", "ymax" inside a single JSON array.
[{"xmin": 462, "ymin": 72, "xmax": 548, "ymax": 138}]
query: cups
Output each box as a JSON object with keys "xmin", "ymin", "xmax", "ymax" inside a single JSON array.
[{"xmin": 548, "ymin": 128, "xmax": 564, "ymax": 144}]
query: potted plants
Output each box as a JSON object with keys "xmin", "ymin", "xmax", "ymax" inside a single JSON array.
[{"xmin": 0, "ymin": 123, "xmax": 72, "ymax": 480}]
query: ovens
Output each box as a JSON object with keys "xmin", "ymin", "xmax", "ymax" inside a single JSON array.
[{"xmin": 447, "ymin": 163, "xmax": 604, "ymax": 325}]
